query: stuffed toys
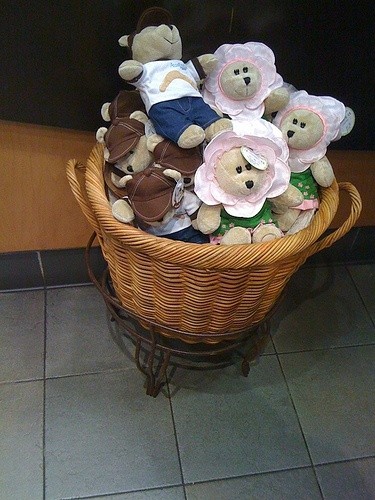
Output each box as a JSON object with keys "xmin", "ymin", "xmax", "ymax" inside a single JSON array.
[
  {"xmin": 146, "ymin": 133, "xmax": 203, "ymax": 194},
  {"xmin": 111, "ymin": 168, "xmax": 210, "ymax": 251},
  {"xmin": 202, "ymin": 41, "xmax": 290, "ymax": 123},
  {"xmin": 117, "ymin": 6, "xmax": 235, "ymax": 150},
  {"xmin": 270, "ymin": 89, "xmax": 355, "ymax": 237},
  {"xmin": 96, "ymin": 110, "xmax": 165, "ymax": 189},
  {"xmin": 193, "ymin": 116, "xmax": 304, "ymax": 247}
]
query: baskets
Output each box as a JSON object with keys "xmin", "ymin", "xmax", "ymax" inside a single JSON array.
[{"xmin": 67, "ymin": 144, "xmax": 363, "ymax": 343}]
